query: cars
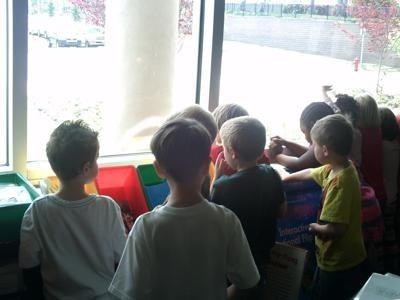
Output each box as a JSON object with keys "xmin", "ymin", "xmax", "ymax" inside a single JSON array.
[
  {"xmin": 78, "ymin": 34, "xmax": 104, "ymax": 48},
  {"xmin": 28, "ymin": 25, "xmax": 81, "ymax": 47}
]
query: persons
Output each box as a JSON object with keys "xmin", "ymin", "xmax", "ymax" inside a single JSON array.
[
  {"xmin": 107, "ymin": 118, "xmax": 261, "ymax": 300},
  {"xmin": 166, "ymin": 104, "xmax": 286, "ymax": 299},
  {"xmin": 268, "ymin": 94, "xmax": 399, "ymax": 299},
  {"xmin": 18, "ymin": 123, "xmax": 127, "ymax": 300}
]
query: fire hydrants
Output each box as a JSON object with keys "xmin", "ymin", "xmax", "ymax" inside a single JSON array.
[{"xmin": 353, "ymin": 56, "xmax": 360, "ymax": 72}]
